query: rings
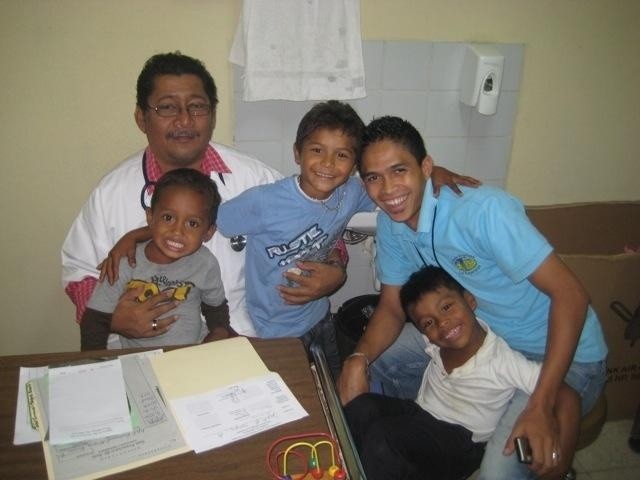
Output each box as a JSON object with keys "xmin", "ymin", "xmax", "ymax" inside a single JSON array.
[
  {"xmin": 152, "ymin": 318, "xmax": 159, "ymax": 331},
  {"xmin": 552, "ymin": 451, "xmax": 561, "ymax": 460}
]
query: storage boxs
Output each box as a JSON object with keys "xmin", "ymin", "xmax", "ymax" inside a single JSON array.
[{"xmin": 523, "ymin": 200, "xmax": 640, "ymax": 421}]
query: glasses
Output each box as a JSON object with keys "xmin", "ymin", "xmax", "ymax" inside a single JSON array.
[{"xmin": 144, "ymin": 99, "xmax": 214, "ymax": 118}]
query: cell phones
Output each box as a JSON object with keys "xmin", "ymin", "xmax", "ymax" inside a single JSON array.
[{"xmin": 515, "ymin": 437, "xmax": 532, "ymax": 464}]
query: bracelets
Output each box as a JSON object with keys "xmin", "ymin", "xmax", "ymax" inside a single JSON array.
[{"xmin": 346, "ymin": 351, "xmax": 370, "ymax": 373}]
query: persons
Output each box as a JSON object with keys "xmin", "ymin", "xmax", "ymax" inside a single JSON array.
[
  {"xmin": 332, "ymin": 114, "xmax": 611, "ymax": 480},
  {"xmin": 79, "ymin": 166, "xmax": 231, "ymax": 356},
  {"xmin": 95, "ymin": 99, "xmax": 483, "ymax": 379},
  {"xmin": 59, "ymin": 50, "xmax": 350, "ymax": 351},
  {"xmin": 340, "ymin": 265, "xmax": 582, "ymax": 480}
]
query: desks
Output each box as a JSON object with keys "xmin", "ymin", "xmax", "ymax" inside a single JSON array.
[{"xmin": 0, "ymin": 338, "xmax": 367, "ymax": 480}]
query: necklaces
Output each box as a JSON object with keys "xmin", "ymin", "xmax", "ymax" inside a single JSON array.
[{"xmin": 318, "ymin": 189, "xmax": 341, "ymax": 212}]
